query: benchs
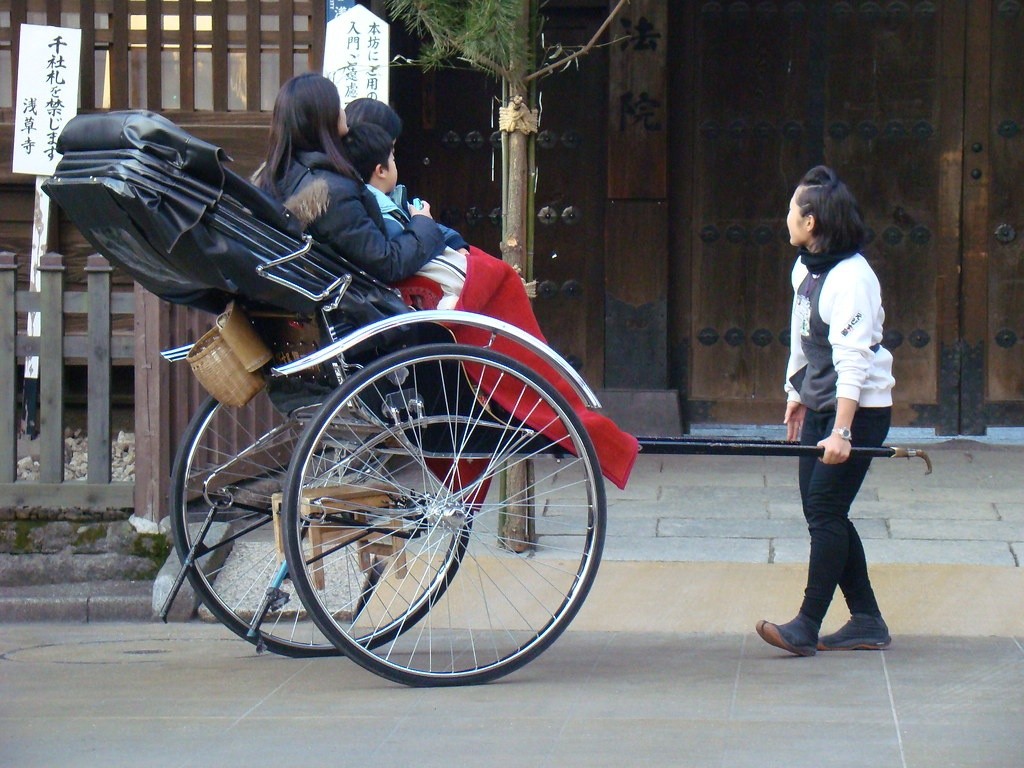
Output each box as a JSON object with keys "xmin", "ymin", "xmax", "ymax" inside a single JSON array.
[{"xmin": 246, "ymin": 304, "xmax": 526, "ymax": 454}]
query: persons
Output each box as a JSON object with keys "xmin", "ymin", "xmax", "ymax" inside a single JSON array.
[
  {"xmin": 756, "ymin": 165, "xmax": 896, "ymax": 656},
  {"xmin": 250, "ymin": 73, "xmax": 471, "ymax": 283}
]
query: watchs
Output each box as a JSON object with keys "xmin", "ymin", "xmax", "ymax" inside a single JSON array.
[{"xmin": 832, "ymin": 427, "xmax": 851, "ymax": 440}]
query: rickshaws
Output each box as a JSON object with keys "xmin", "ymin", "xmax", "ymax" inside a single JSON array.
[{"xmin": 40, "ymin": 106, "xmax": 933, "ymax": 689}]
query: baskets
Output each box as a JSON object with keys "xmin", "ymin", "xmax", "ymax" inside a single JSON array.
[{"xmin": 185, "ymin": 326, "xmax": 268, "ymax": 408}]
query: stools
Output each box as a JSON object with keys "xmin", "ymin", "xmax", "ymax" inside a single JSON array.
[{"xmin": 270, "ymin": 482, "xmax": 407, "ymax": 591}]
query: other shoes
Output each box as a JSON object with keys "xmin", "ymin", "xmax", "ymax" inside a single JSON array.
[
  {"xmin": 756, "ymin": 612, "xmax": 819, "ymax": 656},
  {"xmin": 816, "ymin": 617, "xmax": 892, "ymax": 650}
]
